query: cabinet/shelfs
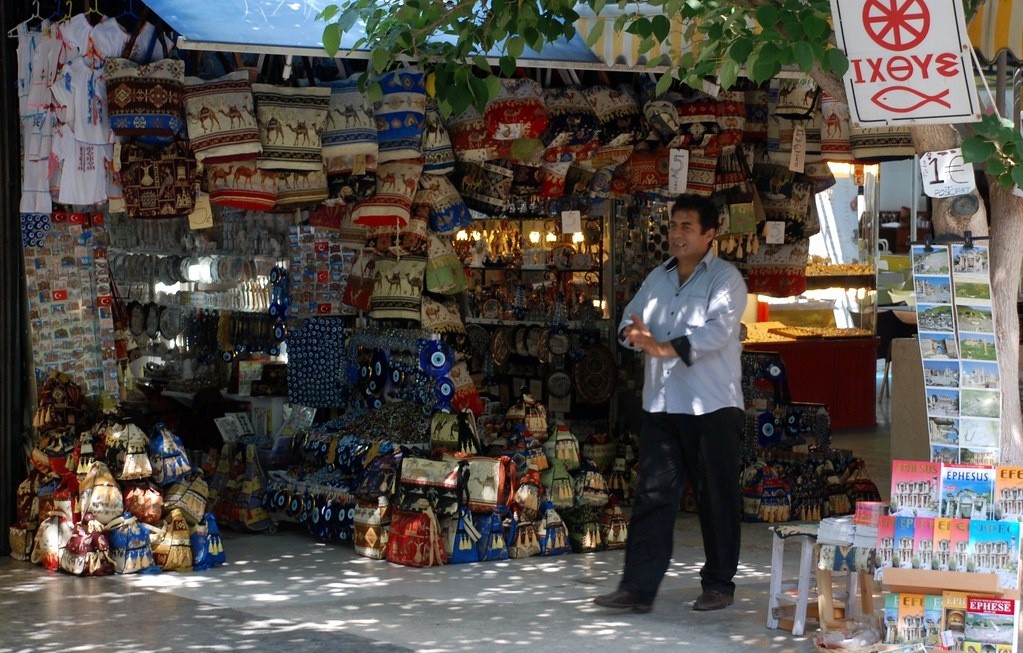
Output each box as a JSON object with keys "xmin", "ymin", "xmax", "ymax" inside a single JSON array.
[{"xmin": 738, "ymin": 273, "xmax": 878, "ymax": 430}]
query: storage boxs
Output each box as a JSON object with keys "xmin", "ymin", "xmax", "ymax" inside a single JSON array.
[
  {"xmin": 880, "ymin": 566, "xmax": 999, "ymax": 595},
  {"xmin": 234, "ymin": 436, "xmax": 291, "ymax": 469}
]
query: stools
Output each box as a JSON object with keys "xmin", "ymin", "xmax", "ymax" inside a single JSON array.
[{"xmin": 768, "ymin": 522, "xmax": 856, "ymax": 635}]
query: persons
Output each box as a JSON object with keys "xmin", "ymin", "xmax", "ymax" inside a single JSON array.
[{"xmin": 593, "ymin": 194, "xmax": 747, "ymax": 613}]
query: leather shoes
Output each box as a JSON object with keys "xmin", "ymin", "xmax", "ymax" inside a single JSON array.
[
  {"xmin": 693, "ymin": 589, "xmax": 735, "ymax": 611},
  {"xmin": 593, "ymin": 589, "xmax": 652, "ymax": 613}
]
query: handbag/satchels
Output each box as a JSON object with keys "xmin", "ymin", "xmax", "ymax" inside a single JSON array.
[
  {"xmin": 740, "ymin": 448, "xmax": 883, "ymax": 523},
  {"xmin": 389, "ymin": 403, "xmax": 630, "ymax": 567},
  {"xmin": 108, "ymin": 22, "xmax": 810, "ymax": 233},
  {"xmin": 341, "ymin": 236, "xmax": 485, "ymax": 414},
  {"xmin": 10, "ymin": 417, "xmax": 224, "ymax": 575}
]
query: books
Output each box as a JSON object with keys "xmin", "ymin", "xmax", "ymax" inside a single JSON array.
[
  {"xmin": 876, "ymin": 461, "xmax": 1023, "ymax": 589},
  {"xmin": 816, "ymin": 502, "xmax": 890, "ymax": 548},
  {"xmin": 882, "ymin": 591, "xmax": 1020, "ymax": 653}
]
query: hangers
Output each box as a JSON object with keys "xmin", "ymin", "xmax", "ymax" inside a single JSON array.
[{"xmin": 4, "ymin": 0, "xmax": 142, "ymax": 39}]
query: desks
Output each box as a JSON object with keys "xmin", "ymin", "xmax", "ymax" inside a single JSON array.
[
  {"xmin": 879, "ymin": 206, "xmax": 931, "ymax": 253},
  {"xmin": 811, "ymin": 542, "xmax": 877, "ymax": 634}
]
